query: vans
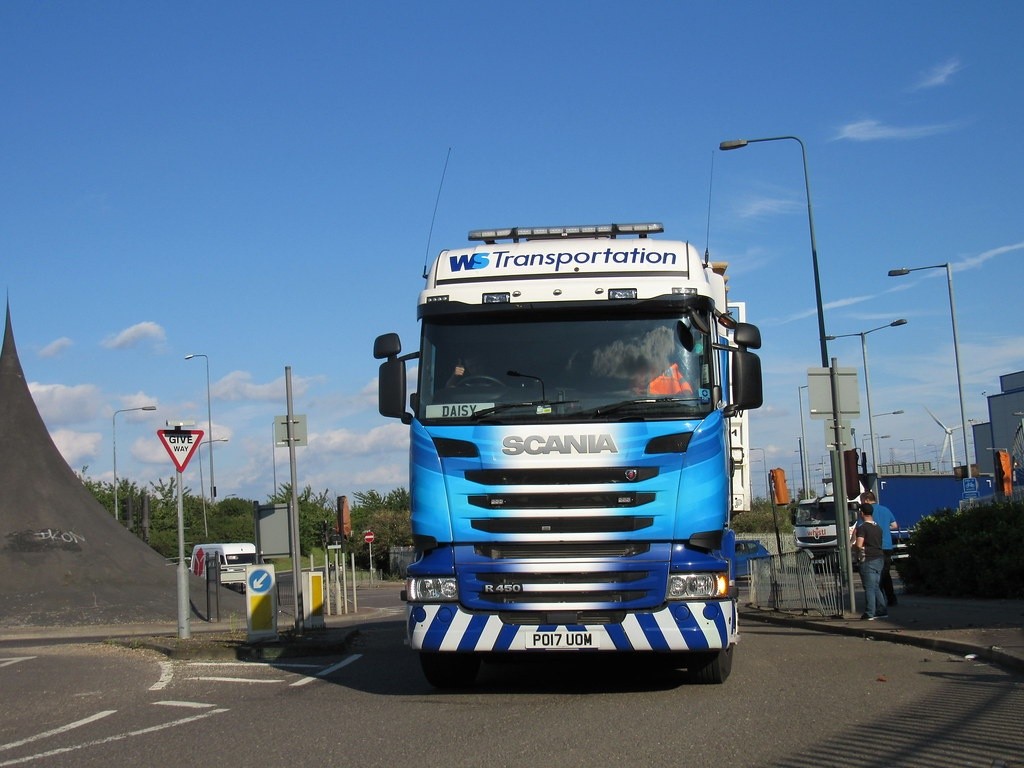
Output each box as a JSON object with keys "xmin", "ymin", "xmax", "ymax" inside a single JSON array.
[{"xmin": 189, "ymin": 542, "xmax": 259, "ymax": 591}]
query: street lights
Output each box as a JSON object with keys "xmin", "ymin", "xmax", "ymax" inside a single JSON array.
[
  {"xmin": 718, "ymin": 135, "xmax": 831, "ymax": 370},
  {"xmin": 198, "ymin": 438, "xmax": 228, "ymax": 542},
  {"xmin": 870, "ymin": 409, "xmax": 905, "ymax": 476},
  {"xmin": 820, "ymin": 318, "xmax": 909, "ymax": 505},
  {"xmin": 888, "ymin": 262, "xmax": 974, "ymax": 479},
  {"xmin": 185, "ymin": 353, "xmax": 215, "ymax": 505},
  {"xmin": 110, "ymin": 405, "xmax": 158, "ymax": 522}
]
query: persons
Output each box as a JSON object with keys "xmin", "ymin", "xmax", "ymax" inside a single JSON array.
[
  {"xmin": 850, "ymin": 491, "xmax": 900, "ymax": 607},
  {"xmin": 854, "ymin": 504, "xmax": 887, "ymax": 620}
]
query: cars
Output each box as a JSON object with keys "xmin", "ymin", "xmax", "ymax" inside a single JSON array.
[{"xmin": 735, "ymin": 539, "xmax": 771, "ymax": 581}]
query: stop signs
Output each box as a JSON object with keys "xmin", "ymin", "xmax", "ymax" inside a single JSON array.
[{"xmin": 364, "ymin": 531, "xmax": 375, "ymax": 543}]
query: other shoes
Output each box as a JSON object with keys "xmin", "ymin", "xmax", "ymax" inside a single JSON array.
[{"xmin": 887, "ymin": 600, "xmax": 897, "ymax": 606}]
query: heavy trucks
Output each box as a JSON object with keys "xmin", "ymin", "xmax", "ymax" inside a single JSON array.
[{"xmin": 372, "ymin": 221, "xmax": 763, "ymax": 683}]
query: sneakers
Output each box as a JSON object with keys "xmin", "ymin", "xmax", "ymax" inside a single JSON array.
[
  {"xmin": 873, "ymin": 610, "xmax": 888, "ymax": 619},
  {"xmin": 860, "ymin": 613, "xmax": 874, "ymax": 621}
]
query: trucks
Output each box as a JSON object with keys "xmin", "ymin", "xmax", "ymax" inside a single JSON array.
[{"xmin": 788, "ymin": 472, "xmax": 995, "ymax": 575}]
